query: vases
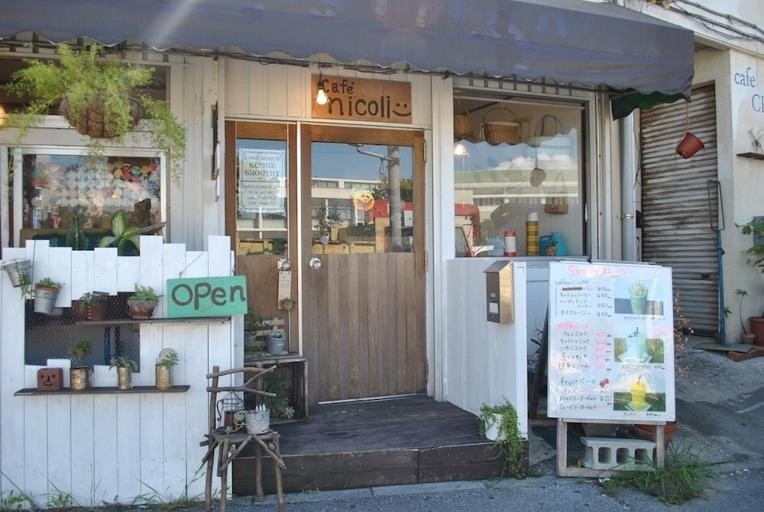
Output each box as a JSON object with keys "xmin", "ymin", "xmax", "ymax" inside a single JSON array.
[{"xmin": 676, "ymin": 130, "xmax": 704, "ymax": 160}]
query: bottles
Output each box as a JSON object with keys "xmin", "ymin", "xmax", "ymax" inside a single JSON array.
[
  {"xmin": 526, "ymin": 210, "xmax": 539, "ymax": 257},
  {"xmin": 506, "ymin": 230, "xmax": 516, "ymax": 257}
]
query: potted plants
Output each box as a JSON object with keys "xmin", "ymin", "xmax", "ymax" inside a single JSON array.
[
  {"xmin": 714, "ymin": 240, "xmax": 764, "ymax": 345},
  {"xmin": 0, "ymin": 39, "xmax": 191, "ymax": 186},
  {"xmin": 0, "ymin": 255, "xmax": 159, "ymax": 320},
  {"xmin": 67, "ymin": 339, "xmax": 180, "ymax": 390},
  {"xmin": 475, "ymin": 397, "xmax": 526, "ymax": 487},
  {"xmin": 218, "ymin": 401, "xmax": 271, "ymax": 436},
  {"xmin": 243, "ymin": 308, "xmax": 299, "ymax": 420}
]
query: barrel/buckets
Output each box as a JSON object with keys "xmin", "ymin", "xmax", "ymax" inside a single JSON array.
[
  {"xmin": 244, "ymin": 408, "xmax": 271, "ymax": 435},
  {"xmin": 265, "ymin": 333, "xmax": 286, "ymax": 355},
  {"xmin": 0, "ymin": 258, "xmax": 32, "ymax": 286},
  {"xmin": 34, "ymin": 287, "xmax": 59, "ymax": 314}
]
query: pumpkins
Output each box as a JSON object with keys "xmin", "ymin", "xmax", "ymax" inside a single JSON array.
[{"xmin": 353, "ymin": 190, "xmax": 374, "ymax": 211}]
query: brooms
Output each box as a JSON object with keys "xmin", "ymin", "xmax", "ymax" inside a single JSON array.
[{"xmin": 279, "ymin": 296, "xmax": 297, "ymax": 355}]
